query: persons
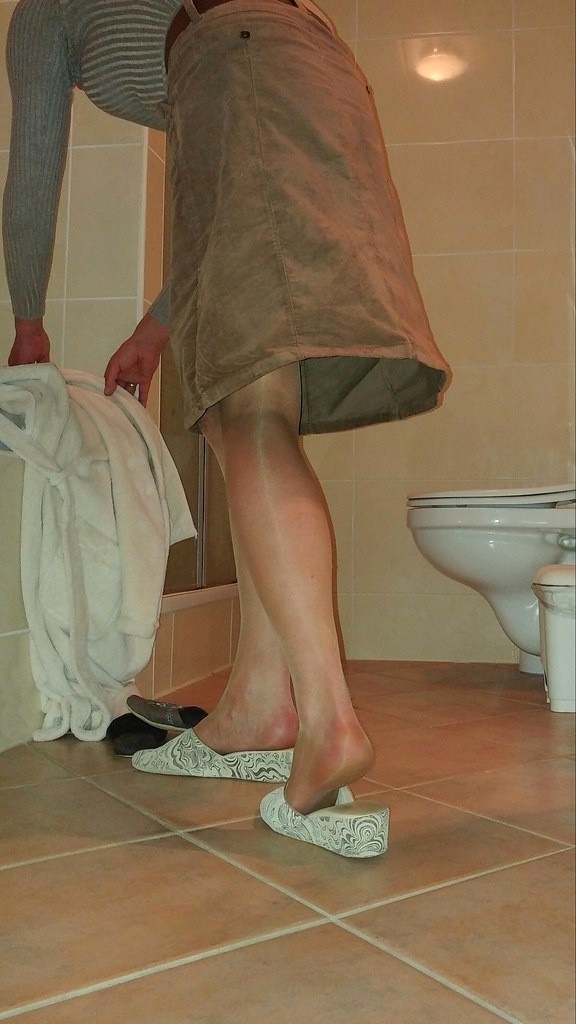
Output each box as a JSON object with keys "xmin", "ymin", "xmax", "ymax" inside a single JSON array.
[{"xmin": 2, "ymin": 0, "xmax": 452, "ymax": 857}]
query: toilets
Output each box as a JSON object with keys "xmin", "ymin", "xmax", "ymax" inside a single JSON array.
[{"xmin": 404, "ymin": 483, "xmax": 576, "ymax": 676}]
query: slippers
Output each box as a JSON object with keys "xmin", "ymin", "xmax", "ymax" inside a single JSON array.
[
  {"xmin": 125, "ymin": 693, "xmax": 211, "ymax": 732},
  {"xmin": 260, "ymin": 784, "xmax": 390, "ymax": 859},
  {"xmin": 106, "ymin": 712, "xmax": 168, "ymax": 759},
  {"xmin": 132, "ymin": 728, "xmax": 294, "ymax": 785}
]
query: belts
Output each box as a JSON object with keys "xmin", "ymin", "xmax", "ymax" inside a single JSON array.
[{"xmin": 162, "ymin": 0, "xmax": 221, "ymax": 72}]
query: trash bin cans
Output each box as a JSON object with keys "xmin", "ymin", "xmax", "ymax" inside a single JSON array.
[{"xmin": 530, "ymin": 564, "xmax": 576, "ymax": 714}]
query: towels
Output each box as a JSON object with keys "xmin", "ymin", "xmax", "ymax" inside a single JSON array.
[{"xmin": 1, "ymin": 362, "xmax": 201, "ymax": 746}]
